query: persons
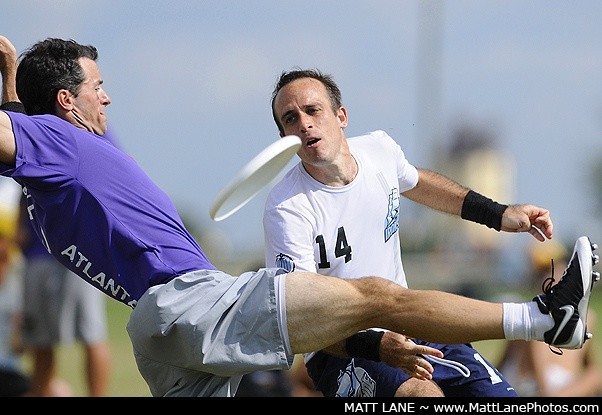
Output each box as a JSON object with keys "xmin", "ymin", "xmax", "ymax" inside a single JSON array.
[
  {"xmin": 17, "ymin": 193, "xmax": 110, "ymax": 396},
  {"xmin": 1, "ymin": 35, "xmax": 600, "ymax": 397},
  {"xmin": 262, "ymin": 67, "xmax": 553, "ymax": 397}
]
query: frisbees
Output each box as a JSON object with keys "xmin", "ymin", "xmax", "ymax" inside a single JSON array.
[{"xmin": 209, "ymin": 134, "xmax": 303, "ymax": 223}]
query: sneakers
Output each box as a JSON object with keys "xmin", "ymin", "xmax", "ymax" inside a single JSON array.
[{"xmin": 534, "ymin": 236, "xmax": 600, "ymax": 355}]
